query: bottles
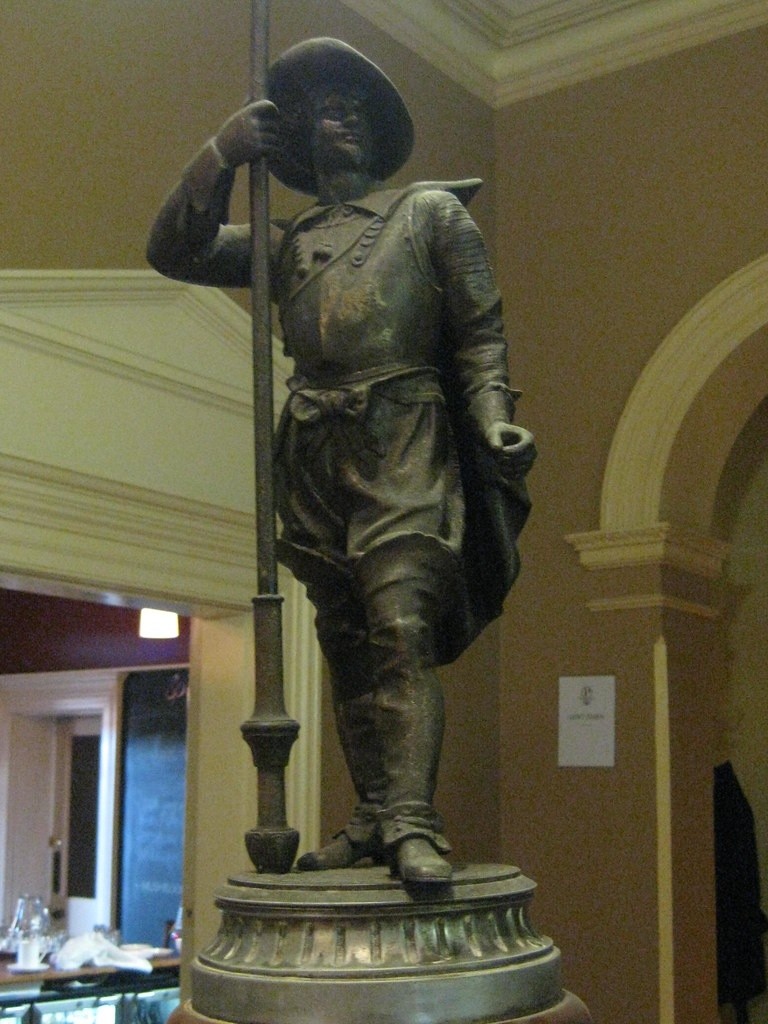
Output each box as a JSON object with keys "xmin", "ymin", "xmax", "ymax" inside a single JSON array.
[{"xmin": 169, "ymin": 906, "xmax": 183, "ymax": 955}]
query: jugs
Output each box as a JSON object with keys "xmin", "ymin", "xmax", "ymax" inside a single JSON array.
[{"xmin": 8, "ymin": 895, "xmax": 51, "ymax": 942}]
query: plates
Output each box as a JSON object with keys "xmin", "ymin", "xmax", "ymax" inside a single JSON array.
[
  {"xmin": 129, "ymin": 947, "xmax": 174, "ymax": 956},
  {"xmin": 121, "ymin": 944, "xmax": 151, "ymax": 949},
  {"xmin": 6, "ymin": 963, "xmax": 50, "ymax": 972}
]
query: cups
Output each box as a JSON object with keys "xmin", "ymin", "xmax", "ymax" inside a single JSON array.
[
  {"xmin": 175, "ymin": 938, "xmax": 183, "ymax": 954},
  {"xmin": 17, "ymin": 941, "xmax": 47, "ymax": 967}
]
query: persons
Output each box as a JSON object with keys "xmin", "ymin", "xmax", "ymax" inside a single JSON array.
[{"xmin": 144, "ymin": 35, "xmax": 539, "ymax": 885}]
